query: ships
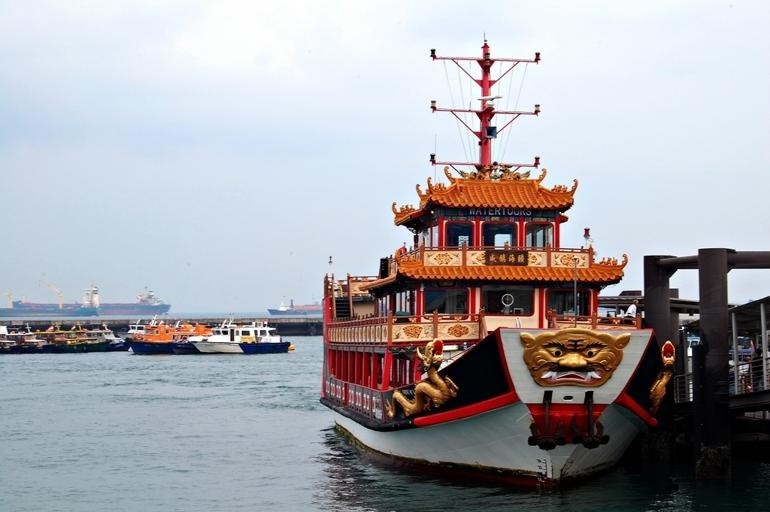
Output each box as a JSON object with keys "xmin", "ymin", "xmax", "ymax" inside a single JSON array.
[{"xmin": 12, "ymin": 287, "xmax": 171, "ymax": 317}]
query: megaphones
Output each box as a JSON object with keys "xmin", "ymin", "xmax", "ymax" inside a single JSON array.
[{"xmin": 485, "ymin": 126, "xmax": 497, "ymax": 139}]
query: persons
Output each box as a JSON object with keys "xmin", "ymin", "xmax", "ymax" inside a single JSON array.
[
  {"xmin": 747, "ymin": 347, "xmax": 764, "ymax": 391},
  {"xmin": 623, "ymin": 299, "xmax": 639, "ymax": 324}
]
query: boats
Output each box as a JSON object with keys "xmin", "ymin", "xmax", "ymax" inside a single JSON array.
[
  {"xmin": 319, "ymin": 32, "xmax": 677, "ymax": 489},
  {"xmin": 0, "ymin": 324, "xmax": 295, "ymax": 354},
  {"xmin": 267, "ymin": 296, "xmax": 323, "ymax": 315}
]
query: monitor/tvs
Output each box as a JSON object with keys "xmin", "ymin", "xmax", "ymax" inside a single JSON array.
[{"xmin": 513, "ymin": 307, "xmax": 524, "ymax": 315}]
